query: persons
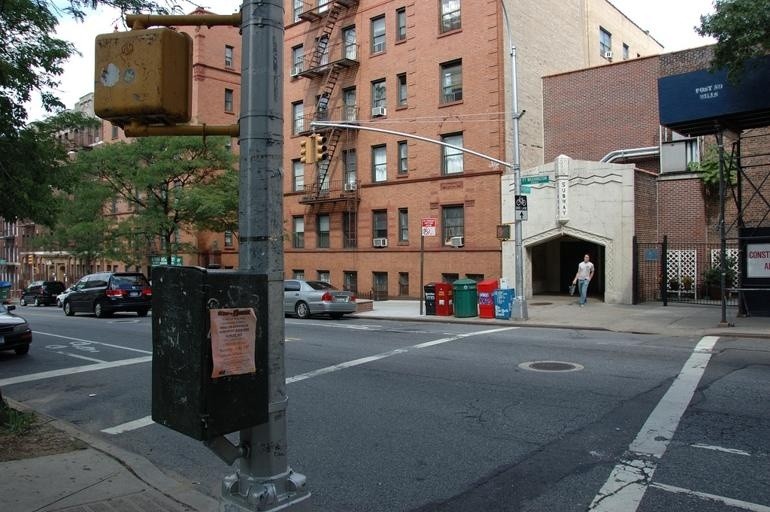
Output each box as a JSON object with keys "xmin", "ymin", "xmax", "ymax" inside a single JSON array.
[{"xmin": 572, "ymin": 253, "xmax": 595, "ymax": 307}]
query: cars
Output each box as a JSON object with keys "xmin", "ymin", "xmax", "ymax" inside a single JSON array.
[
  {"xmin": 284, "ymin": 280, "xmax": 356, "ymax": 319},
  {"xmin": 0, "ymin": 273, "xmax": 153, "ymax": 354}
]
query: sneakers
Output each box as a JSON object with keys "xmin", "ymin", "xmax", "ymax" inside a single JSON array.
[{"xmin": 579, "ymin": 301, "xmax": 588, "ymax": 308}]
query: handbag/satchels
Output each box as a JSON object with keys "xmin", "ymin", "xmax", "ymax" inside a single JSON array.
[{"xmin": 569, "ymin": 284, "xmax": 576, "ymax": 296}]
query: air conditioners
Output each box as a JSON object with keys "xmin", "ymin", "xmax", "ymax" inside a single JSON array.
[
  {"xmin": 451, "ymin": 237, "xmax": 464, "ymax": 247},
  {"xmin": 371, "ymin": 107, "xmax": 386, "ymax": 116},
  {"xmin": 344, "ymin": 184, "xmax": 357, "ymax": 191},
  {"xmin": 373, "ymin": 239, "xmax": 387, "ymax": 248},
  {"xmin": 605, "ymin": 51, "xmax": 613, "ymax": 59}
]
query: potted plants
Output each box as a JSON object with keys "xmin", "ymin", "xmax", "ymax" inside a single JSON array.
[
  {"xmin": 682, "ymin": 274, "xmax": 693, "ymax": 291},
  {"xmin": 669, "ymin": 277, "xmax": 678, "ymax": 290}
]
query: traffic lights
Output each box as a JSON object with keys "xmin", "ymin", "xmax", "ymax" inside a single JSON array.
[{"xmin": 299, "ymin": 134, "xmax": 327, "ymax": 164}]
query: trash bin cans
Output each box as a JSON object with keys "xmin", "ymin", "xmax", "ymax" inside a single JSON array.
[
  {"xmin": 452, "ymin": 277, "xmax": 478, "ymax": 317},
  {"xmin": 0, "ymin": 280, "xmax": 12, "ymax": 299}
]
